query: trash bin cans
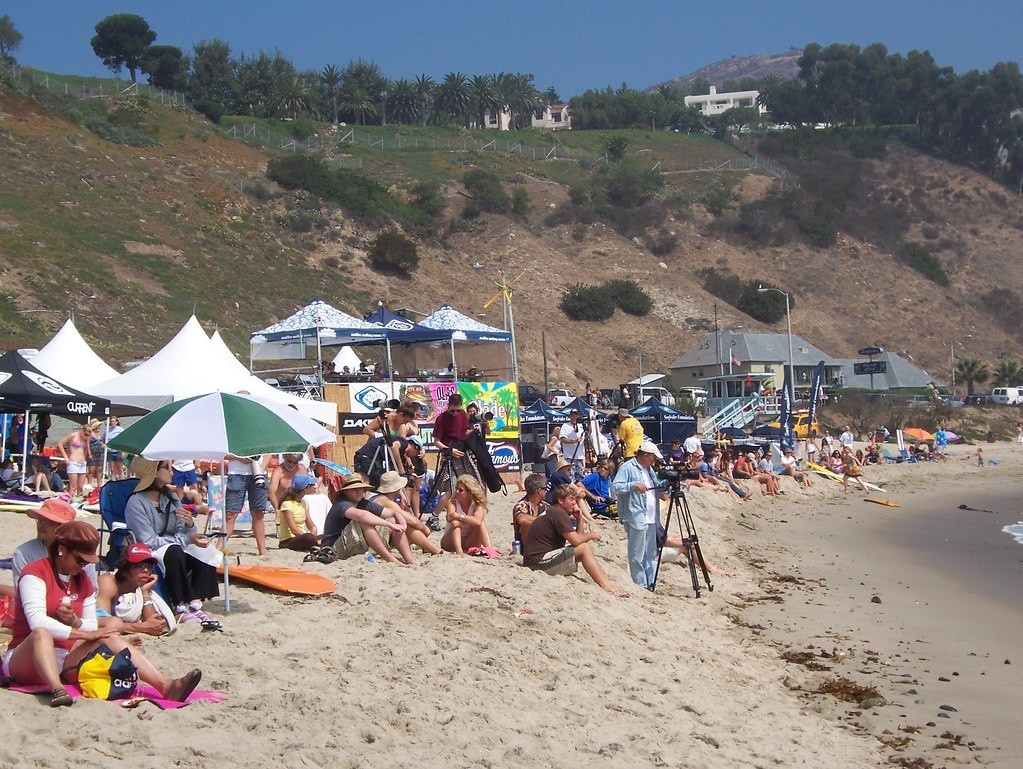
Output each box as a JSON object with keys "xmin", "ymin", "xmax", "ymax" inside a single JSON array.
[
  {"xmin": 988, "ymin": 431, "xmax": 995, "ymax": 443},
  {"xmin": 876, "ymin": 428, "xmax": 885, "ymax": 443}
]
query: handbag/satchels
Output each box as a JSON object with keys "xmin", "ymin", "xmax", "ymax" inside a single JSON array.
[{"xmin": 58, "ymin": 643, "xmax": 137, "ymax": 701}]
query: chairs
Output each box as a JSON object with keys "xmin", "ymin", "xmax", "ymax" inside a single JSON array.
[
  {"xmin": 264, "ymin": 372, "xmax": 325, "ymax": 401},
  {"xmin": 0, "ymin": 473, "xmax": 23, "ymax": 489},
  {"xmin": 97, "ymin": 478, "xmax": 227, "ymax": 611}
]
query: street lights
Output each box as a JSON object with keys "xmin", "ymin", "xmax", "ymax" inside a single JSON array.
[
  {"xmin": 950, "ymin": 335, "xmax": 972, "ymax": 396},
  {"xmin": 757, "ymin": 284, "xmax": 794, "ymax": 401},
  {"xmin": 718, "ymin": 326, "xmax": 743, "ymax": 375}
]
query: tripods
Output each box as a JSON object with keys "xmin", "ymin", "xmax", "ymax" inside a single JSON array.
[
  {"xmin": 642, "ymin": 480, "xmax": 714, "ymax": 600},
  {"xmin": 419, "ymin": 448, "xmax": 460, "ymax": 521},
  {"xmin": 570, "ymin": 422, "xmax": 602, "ymax": 474},
  {"xmin": 608, "ymin": 439, "xmax": 626, "ymax": 460},
  {"xmin": 366, "ymin": 409, "xmax": 414, "ymax": 516}
]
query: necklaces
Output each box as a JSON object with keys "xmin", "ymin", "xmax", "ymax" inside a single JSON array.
[{"xmin": 63, "ymin": 579, "xmax": 72, "ymax": 595}]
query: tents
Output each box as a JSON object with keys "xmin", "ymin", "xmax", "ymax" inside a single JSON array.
[
  {"xmin": 0, "ymin": 311, "xmax": 344, "ymax": 481},
  {"xmin": 358, "ymin": 300, "xmax": 464, "ymax": 378},
  {"xmin": 249, "ymin": 301, "xmax": 398, "ymax": 383},
  {"xmin": 418, "ymin": 300, "xmax": 519, "ymax": 380}
]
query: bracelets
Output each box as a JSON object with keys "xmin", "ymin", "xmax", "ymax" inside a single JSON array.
[
  {"xmin": 72, "ymin": 612, "xmax": 77, "ymax": 624},
  {"xmin": 144, "ymin": 601, "xmax": 152, "ymax": 605},
  {"xmin": 460, "ymin": 515, "xmax": 464, "ymax": 519},
  {"xmin": 143, "ymin": 595, "xmax": 150, "ymax": 598}
]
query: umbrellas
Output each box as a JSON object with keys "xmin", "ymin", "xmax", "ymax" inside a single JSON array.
[
  {"xmin": 902, "ymin": 427, "xmax": 935, "ymax": 444},
  {"xmin": 932, "ymin": 429, "xmax": 960, "ymax": 442},
  {"xmin": 106, "ymin": 387, "xmax": 339, "ymax": 611}
]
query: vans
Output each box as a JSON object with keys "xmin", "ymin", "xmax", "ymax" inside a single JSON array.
[{"xmin": 992, "ymin": 386, "xmax": 1023, "ymax": 405}]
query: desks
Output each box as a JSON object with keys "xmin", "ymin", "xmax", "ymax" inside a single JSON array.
[{"xmin": 324, "ymin": 374, "xmax": 498, "ymax": 382}]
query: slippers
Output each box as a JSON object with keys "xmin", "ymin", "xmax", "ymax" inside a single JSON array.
[{"xmin": 200, "ymin": 621, "xmax": 219, "ymax": 629}]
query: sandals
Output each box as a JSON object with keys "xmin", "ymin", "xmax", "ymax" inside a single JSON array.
[
  {"xmin": 304, "ymin": 546, "xmax": 322, "ymax": 562},
  {"xmin": 317, "ymin": 546, "xmax": 339, "ymax": 563}
]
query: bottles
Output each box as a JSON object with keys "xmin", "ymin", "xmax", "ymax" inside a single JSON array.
[
  {"xmin": 43, "ymin": 447, "xmax": 56, "ymax": 456},
  {"xmin": 512, "ymin": 539, "xmax": 520, "ymax": 554},
  {"xmin": 365, "ymin": 551, "xmax": 378, "ymax": 563}
]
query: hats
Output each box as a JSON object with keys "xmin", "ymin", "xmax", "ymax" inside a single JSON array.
[
  {"xmin": 617, "ymin": 408, "xmax": 631, "ymax": 418},
  {"xmin": 747, "ymin": 453, "xmax": 756, "ymax": 461},
  {"xmin": 570, "ymin": 408, "xmax": 579, "ymax": 415},
  {"xmin": 409, "ymin": 434, "xmax": 423, "ymax": 450},
  {"xmin": 26, "ymin": 499, "xmax": 159, "ymax": 567},
  {"xmin": 130, "ymin": 456, "xmax": 163, "ymax": 492},
  {"xmin": 554, "ymin": 459, "xmax": 574, "ymax": 471},
  {"xmin": 638, "ymin": 441, "xmax": 664, "ymax": 459},
  {"xmin": 291, "ymin": 471, "xmax": 411, "ymax": 498},
  {"xmin": 89, "ymin": 418, "xmax": 102, "ymax": 429}
]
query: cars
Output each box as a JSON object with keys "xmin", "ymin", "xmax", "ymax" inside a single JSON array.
[
  {"xmin": 713, "ymin": 426, "xmax": 782, "ymax": 439},
  {"xmin": 903, "ymin": 394, "xmax": 965, "ymax": 408}
]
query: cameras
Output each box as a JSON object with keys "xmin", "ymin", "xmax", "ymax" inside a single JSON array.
[
  {"xmin": 576, "ymin": 415, "xmax": 598, "ymax": 423},
  {"xmin": 250, "ymin": 455, "xmax": 265, "ymax": 488},
  {"xmin": 373, "ymin": 399, "xmax": 400, "ymax": 409},
  {"xmin": 656, "ymin": 469, "xmax": 700, "ymax": 480},
  {"xmin": 469, "ymin": 412, "xmax": 493, "ymax": 424}
]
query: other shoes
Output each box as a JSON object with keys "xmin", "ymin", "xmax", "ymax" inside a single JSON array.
[
  {"xmin": 50, "ymin": 687, "xmax": 73, "ymax": 707},
  {"xmin": 426, "ymin": 517, "xmax": 441, "ymax": 531},
  {"xmin": 470, "ymin": 550, "xmax": 490, "ymax": 558},
  {"xmin": 164, "ymin": 668, "xmax": 202, "ymax": 702}
]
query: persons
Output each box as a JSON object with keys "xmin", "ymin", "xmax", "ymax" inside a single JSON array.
[
  {"xmin": 12, "ymin": 497, "xmax": 142, "ymax": 645},
  {"xmin": 667, "ymin": 422, "xmax": 878, "ymax": 495},
  {"xmin": 909, "ymin": 441, "xmax": 930, "ymax": 461},
  {"xmin": 1015, "ymin": 422, "xmax": 1023, "ymax": 445},
  {"xmin": 972, "ymin": 447, "xmax": 985, "ymax": 468},
  {"xmin": 0, "ymin": 407, "xmax": 209, "ymax": 496},
  {"xmin": 2, "ymin": 519, "xmax": 202, "ymax": 707},
  {"xmin": 511, "ymin": 386, "xmax": 711, "ymax": 589},
  {"xmin": 0, "ymin": 541, "xmax": 167, "ymax": 636},
  {"xmin": 124, "ymin": 455, "xmax": 221, "ymax": 624},
  {"xmin": 269, "ymin": 393, "xmax": 487, "ymax": 564},
  {"xmin": 214, "ymin": 453, "xmax": 270, "ymax": 557},
  {"xmin": 322, "ymin": 358, "xmax": 479, "ymax": 383}
]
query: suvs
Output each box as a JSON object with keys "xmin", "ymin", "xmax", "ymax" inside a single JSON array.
[
  {"xmin": 599, "ymin": 389, "xmax": 621, "ymax": 406},
  {"xmin": 547, "ymin": 389, "xmax": 577, "ymax": 406},
  {"xmin": 768, "ymin": 410, "xmax": 819, "ymax": 437},
  {"xmin": 518, "ymin": 385, "xmax": 547, "ymax": 405}
]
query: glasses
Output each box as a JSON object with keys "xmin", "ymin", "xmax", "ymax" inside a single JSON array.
[
  {"xmin": 158, "ymin": 463, "xmax": 170, "ymax": 471},
  {"xmin": 537, "ymin": 486, "xmax": 548, "ymax": 491},
  {"xmin": 130, "ymin": 561, "xmax": 155, "ymax": 572},
  {"xmin": 786, "ymin": 451, "xmax": 793, "ymax": 453},
  {"xmin": 676, "ymin": 442, "xmax": 681, "ymax": 446},
  {"xmin": 709, "ymin": 456, "xmax": 714, "ymax": 459},
  {"xmin": 455, "ymin": 487, "xmax": 469, "ymax": 493},
  {"xmin": 70, "ymin": 552, "xmax": 90, "ymax": 568},
  {"xmin": 84, "ymin": 431, "xmax": 89, "ymax": 436},
  {"xmin": 449, "ymin": 407, "xmax": 462, "ymax": 413}
]
